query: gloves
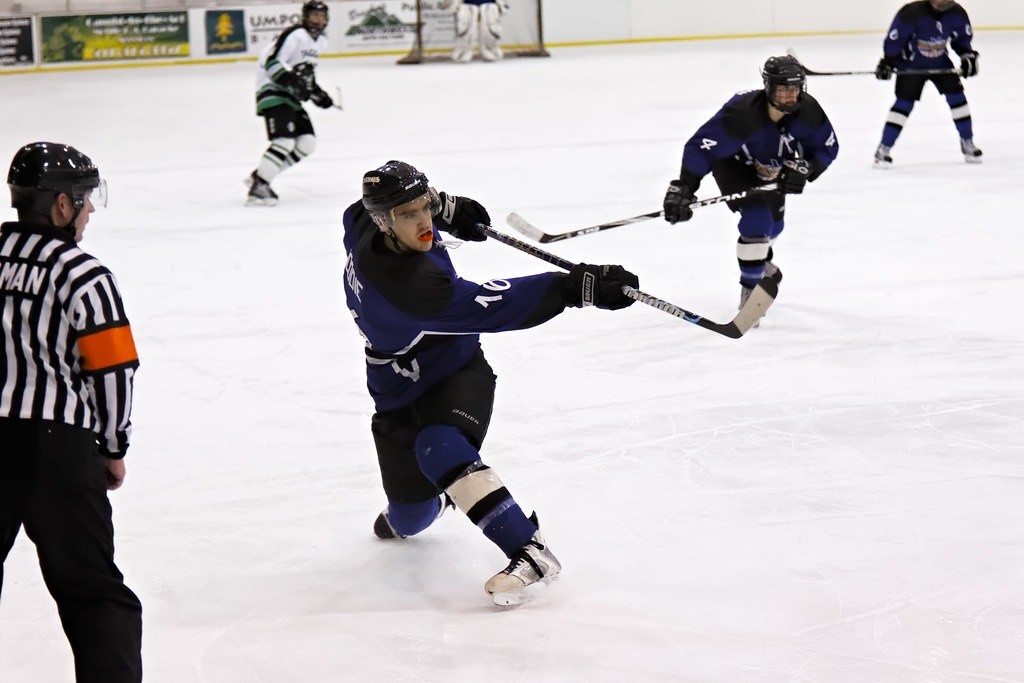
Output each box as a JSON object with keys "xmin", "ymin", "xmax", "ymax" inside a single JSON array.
[
  {"xmin": 434, "ymin": 190, "xmax": 490, "ymax": 243},
  {"xmin": 566, "ymin": 261, "xmax": 640, "ymax": 311},
  {"xmin": 960, "ymin": 51, "xmax": 979, "ymax": 78},
  {"xmin": 778, "ymin": 157, "xmax": 812, "ymax": 195},
  {"xmin": 277, "ymin": 69, "xmax": 308, "ymax": 102},
  {"xmin": 309, "ymin": 82, "xmax": 333, "ymax": 109},
  {"xmin": 663, "ymin": 180, "xmax": 697, "ymax": 225},
  {"xmin": 875, "ymin": 59, "xmax": 893, "ymax": 80}
]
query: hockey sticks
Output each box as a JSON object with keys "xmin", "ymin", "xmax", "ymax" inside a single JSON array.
[
  {"xmin": 506, "ymin": 181, "xmax": 778, "ymax": 244},
  {"xmin": 477, "ymin": 222, "xmax": 778, "ymax": 339},
  {"xmin": 785, "ymin": 46, "xmax": 964, "ymax": 76},
  {"xmin": 310, "ymin": 87, "xmax": 344, "ymax": 111}
]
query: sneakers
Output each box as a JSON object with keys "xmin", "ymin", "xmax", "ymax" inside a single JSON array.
[
  {"xmin": 244, "ymin": 169, "xmax": 280, "ymax": 207},
  {"xmin": 738, "ymin": 257, "xmax": 791, "ymax": 328},
  {"xmin": 874, "ymin": 140, "xmax": 894, "ymax": 170},
  {"xmin": 961, "ymin": 134, "xmax": 986, "ymax": 164},
  {"xmin": 484, "ymin": 511, "xmax": 562, "ymax": 608},
  {"xmin": 373, "ymin": 492, "xmax": 462, "ymax": 539}
]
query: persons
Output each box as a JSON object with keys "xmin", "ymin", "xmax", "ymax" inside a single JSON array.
[
  {"xmin": 873, "ymin": 0, "xmax": 983, "ymax": 168},
  {"xmin": 664, "ymin": 55, "xmax": 839, "ymax": 328},
  {"xmin": 343, "ymin": 160, "xmax": 639, "ymax": 606},
  {"xmin": 438, "ymin": 0, "xmax": 508, "ymax": 62},
  {"xmin": 0, "ymin": 142, "xmax": 143, "ymax": 683},
  {"xmin": 244, "ymin": 1, "xmax": 334, "ymax": 206}
]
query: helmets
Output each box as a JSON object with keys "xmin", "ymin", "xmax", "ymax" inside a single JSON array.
[
  {"xmin": 362, "ymin": 161, "xmax": 429, "ymax": 219},
  {"xmin": 302, "ymin": 0, "xmax": 330, "ymax": 24},
  {"xmin": 762, "ymin": 55, "xmax": 810, "ymax": 115},
  {"xmin": 8, "ymin": 141, "xmax": 99, "ymax": 208}
]
